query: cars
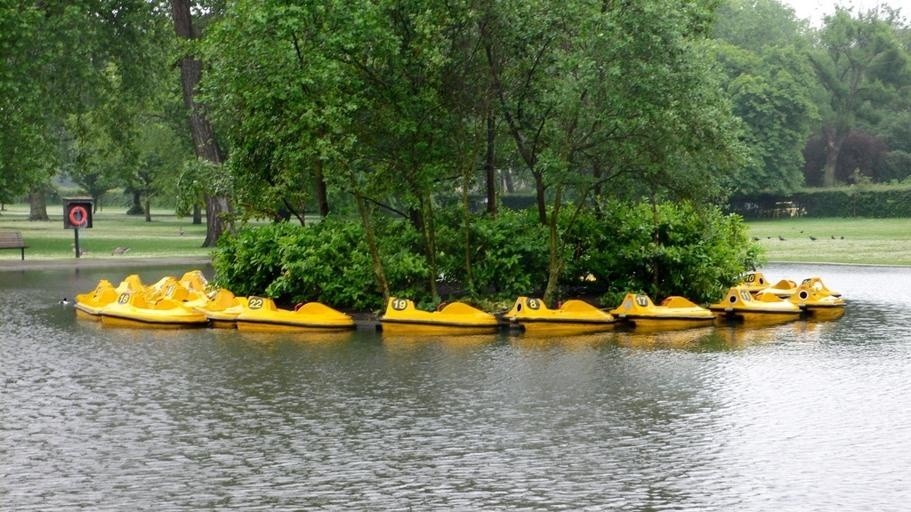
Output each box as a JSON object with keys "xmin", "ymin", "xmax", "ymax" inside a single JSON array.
[{"xmin": 772, "ymin": 200, "xmax": 807, "ymax": 217}]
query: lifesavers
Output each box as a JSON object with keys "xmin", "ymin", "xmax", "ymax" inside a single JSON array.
[{"xmin": 69, "ymin": 206, "xmax": 87, "ymax": 226}]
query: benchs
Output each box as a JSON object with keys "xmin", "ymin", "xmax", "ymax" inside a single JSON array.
[{"xmin": 0, "ymin": 231, "xmax": 31, "ymax": 261}]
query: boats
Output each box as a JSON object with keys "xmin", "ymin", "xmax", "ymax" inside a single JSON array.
[
  {"xmin": 708, "ymin": 272, "xmax": 846, "ymax": 331},
  {"xmin": 369, "ymin": 295, "xmax": 504, "ymax": 332},
  {"xmin": 66, "ymin": 267, "xmax": 357, "ymax": 336},
  {"xmin": 501, "ymin": 292, "xmax": 619, "ymax": 331},
  {"xmin": 609, "ymin": 290, "xmax": 718, "ymax": 332}
]
